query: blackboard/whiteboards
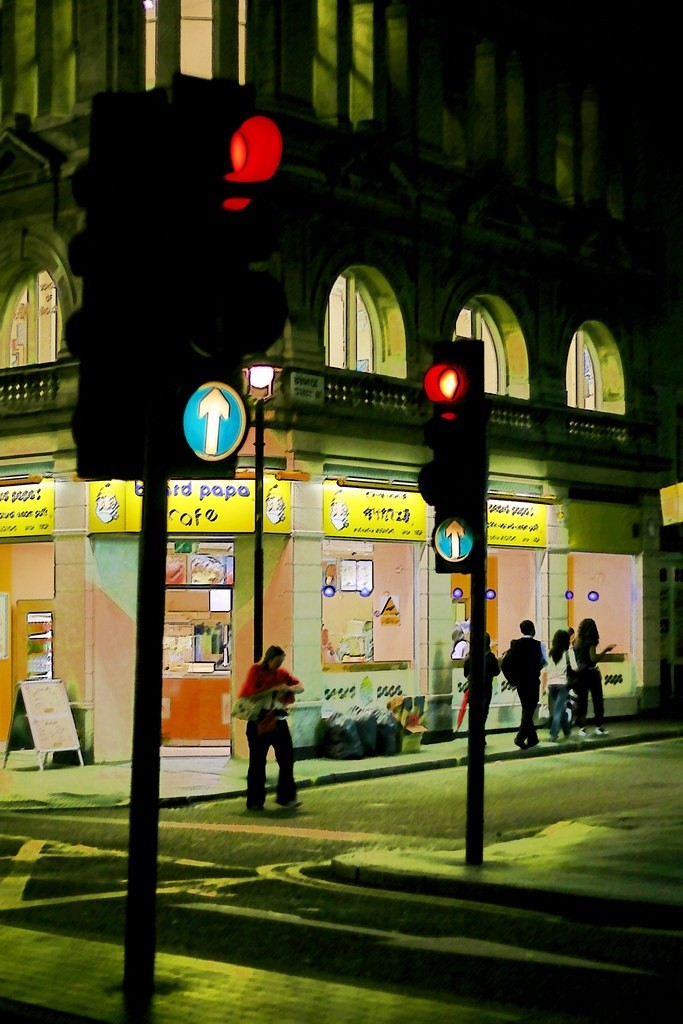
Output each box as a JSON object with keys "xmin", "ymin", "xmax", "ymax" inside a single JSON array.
[{"xmin": 4, "ymin": 679, "xmax": 81, "ymax": 754}]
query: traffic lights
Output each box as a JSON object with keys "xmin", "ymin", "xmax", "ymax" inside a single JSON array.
[
  {"xmin": 164, "ymin": 73, "xmax": 298, "ymax": 372},
  {"xmin": 64, "ymin": 88, "xmax": 156, "ymax": 480},
  {"xmin": 416, "ymin": 338, "xmax": 492, "ymax": 515}
]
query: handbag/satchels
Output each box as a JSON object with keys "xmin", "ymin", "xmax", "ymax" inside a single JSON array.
[{"xmin": 232, "ymin": 695, "xmax": 264, "ymax": 722}]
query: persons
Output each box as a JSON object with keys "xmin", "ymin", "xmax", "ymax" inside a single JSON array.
[
  {"xmin": 238, "ymin": 645, "xmax": 305, "ymax": 810},
  {"xmin": 451, "ymin": 617, "xmax": 617, "ymax": 751},
  {"xmin": 321, "ymin": 627, "xmax": 340, "ymax": 664}
]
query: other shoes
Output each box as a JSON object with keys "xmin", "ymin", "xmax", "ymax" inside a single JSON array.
[
  {"xmin": 580, "ymin": 729, "xmax": 587, "ymax": 738},
  {"xmin": 285, "ymin": 799, "xmax": 300, "ymax": 808},
  {"xmin": 514, "ymin": 737, "xmax": 527, "ymax": 751},
  {"xmin": 595, "ymin": 728, "xmax": 605, "ymax": 736},
  {"xmin": 252, "ymin": 805, "xmax": 264, "ymax": 812}
]
